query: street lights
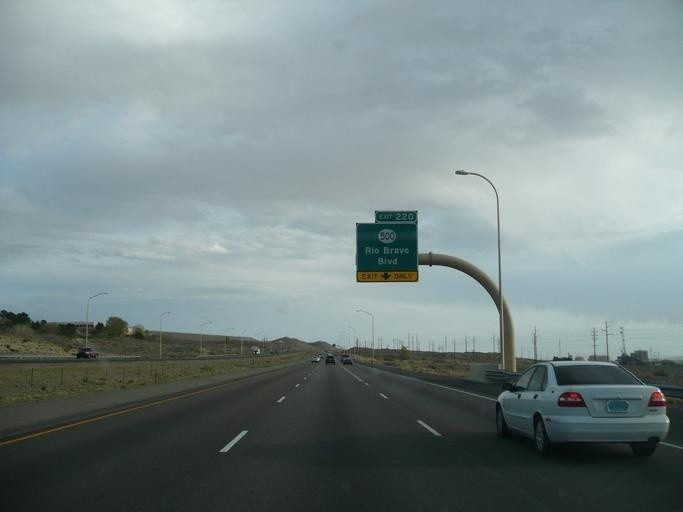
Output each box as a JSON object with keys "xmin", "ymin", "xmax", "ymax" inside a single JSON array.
[
  {"xmin": 455, "ymin": 170, "xmax": 506, "ymax": 374},
  {"xmin": 200, "ymin": 321, "xmax": 248, "ymax": 358},
  {"xmin": 356, "ymin": 309, "xmax": 376, "ymax": 366},
  {"xmin": 339, "ymin": 327, "xmax": 357, "ymax": 355},
  {"xmin": 86, "ymin": 291, "xmax": 107, "ymax": 348},
  {"xmin": 159, "ymin": 311, "xmax": 171, "ymax": 360}
]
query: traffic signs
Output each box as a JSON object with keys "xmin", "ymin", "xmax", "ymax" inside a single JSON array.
[
  {"xmin": 355, "ymin": 222, "xmax": 416, "ymax": 283},
  {"xmin": 374, "ymin": 210, "xmax": 417, "ymax": 222}
]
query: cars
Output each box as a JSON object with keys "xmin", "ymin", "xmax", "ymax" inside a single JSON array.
[
  {"xmin": 311, "ymin": 350, "xmax": 353, "ymax": 367},
  {"xmin": 494, "ymin": 359, "xmax": 671, "ymax": 457},
  {"xmin": 77, "ymin": 347, "xmax": 99, "ymax": 359}
]
